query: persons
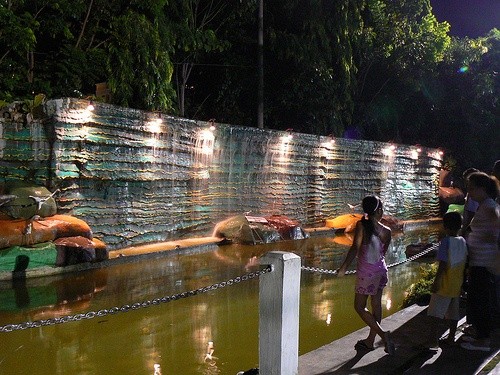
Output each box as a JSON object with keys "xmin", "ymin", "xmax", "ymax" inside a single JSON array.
[
  {"xmin": 460, "ymin": 160, "xmax": 500, "ymax": 351},
  {"xmin": 336, "ymin": 196, "xmax": 395, "ymax": 356},
  {"xmin": 423, "ymin": 212, "xmax": 468, "ymax": 349}
]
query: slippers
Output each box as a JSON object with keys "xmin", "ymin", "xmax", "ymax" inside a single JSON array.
[
  {"xmin": 460, "ymin": 327, "xmax": 489, "ymax": 338},
  {"xmin": 382, "ymin": 330, "xmax": 395, "ymax": 357},
  {"xmin": 354, "ymin": 339, "xmax": 376, "ymax": 351},
  {"xmin": 415, "ymin": 343, "xmax": 437, "ymax": 352},
  {"xmin": 438, "ymin": 338, "xmax": 457, "ymax": 346}
]
